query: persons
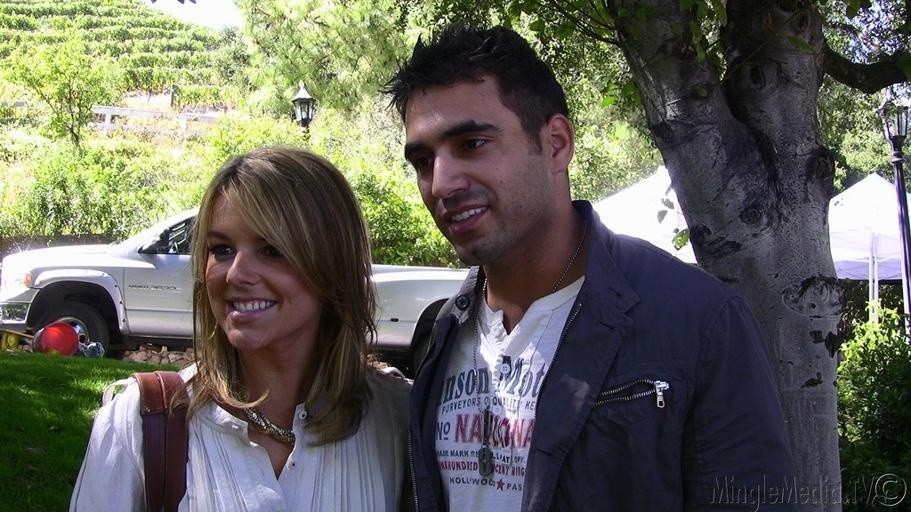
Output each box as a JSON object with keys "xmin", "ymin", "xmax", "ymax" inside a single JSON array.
[
  {"xmin": 69, "ymin": 146, "xmax": 412, "ymax": 511},
  {"xmin": 379, "ymin": 19, "xmax": 800, "ymax": 512}
]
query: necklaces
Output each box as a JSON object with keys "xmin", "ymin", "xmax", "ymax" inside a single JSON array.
[
  {"xmin": 474, "ymin": 220, "xmax": 589, "ymax": 476},
  {"xmin": 242, "ymin": 405, "xmax": 295, "ymax": 444}
]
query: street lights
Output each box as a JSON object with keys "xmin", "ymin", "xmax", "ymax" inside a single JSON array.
[
  {"xmin": 292, "ymin": 81, "xmax": 317, "ymax": 150},
  {"xmin": 875, "ymin": 94, "xmax": 911, "ymax": 319}
]
